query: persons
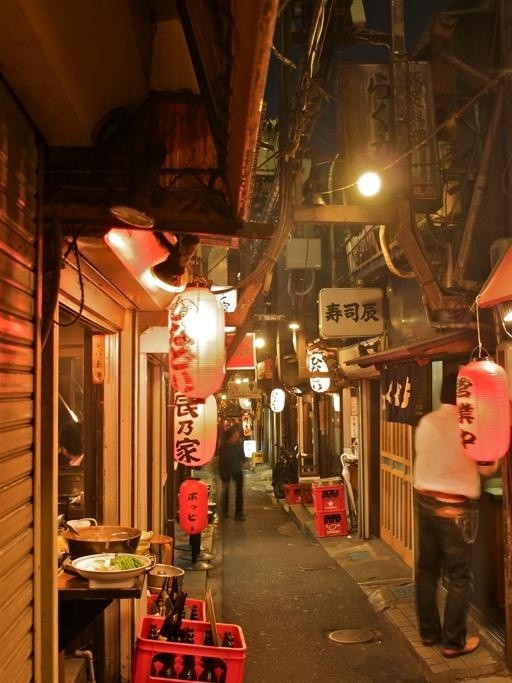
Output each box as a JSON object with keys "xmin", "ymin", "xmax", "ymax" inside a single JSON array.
[
  {"xmin": 216, "ymin": 424, "xmax": 249, "ymax": 521},
  {"xmin": 414, "ymin": 373, "xmax": 501, "ymax": 659},
  {"xmin": 59, "ymin": 421, "xmax": 88, "ymax": 465}
]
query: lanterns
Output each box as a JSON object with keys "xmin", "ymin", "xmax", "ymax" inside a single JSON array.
[
  {"xmin": 311, "ymin": 352, "xmax": 330, "ymax": 393},
  {"xmin": 268, "ymin": 387, "xmax": 287, "ymax": 413},
  {"xmin": 170, "ymin": 387, "xmax": 220, "ymax": 471},
  {"xmin": 455, "ymin": 356, "xmax": 509, "ymax": 467},
  {"xmin": 167, "ymin": 277, "xmax": 228, "ymax": 402},
  {"xmin": 178, "ymin": 474, "xmax": 210, "ymax": 533}
]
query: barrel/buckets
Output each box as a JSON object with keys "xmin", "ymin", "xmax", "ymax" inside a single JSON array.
[
  {"xmin": 148, "ymin": 561, "xmax": 185, "ymax": 598},
  {"xmin": 151, "ymin": 534, "xmax": 174, "ymax": 565}
]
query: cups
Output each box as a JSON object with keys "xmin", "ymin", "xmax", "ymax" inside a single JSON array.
[{"xmin": 57, "ymin": 526, "xmax": 64, "ymax": 544}]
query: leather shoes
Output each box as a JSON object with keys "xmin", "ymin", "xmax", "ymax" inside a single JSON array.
[
  {"xmin": 441, "ymin": 636, "xmax": 480, "ymax": 658},
  {"xmin": 422, "ymin": 625, "xmax": 442, "ymax": 645}
]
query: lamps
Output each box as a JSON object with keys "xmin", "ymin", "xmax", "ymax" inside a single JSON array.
[
  {"xmin": 149, "ymin": 231, "xmax": 200, "ymax": 293},
  {"xmin": 500, "ymin": 303, "xmax": 512, "ymax": 321},
  {"xmin": 108, "ymin": 144, "xmax": 168, "ymax": 229}
]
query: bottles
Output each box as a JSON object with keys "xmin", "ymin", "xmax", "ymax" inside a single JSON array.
[
  {"xmin": 321, "ymin": 490, "xmax": 341, "ymax": 524},
  {"xmin": 148, "ymin": 576, "xmax": 234, "ymax": 682}
]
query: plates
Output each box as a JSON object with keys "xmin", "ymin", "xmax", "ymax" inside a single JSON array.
[{"xmin": 135, "ymin": 530, "xmax": 153, "ymax": 553}]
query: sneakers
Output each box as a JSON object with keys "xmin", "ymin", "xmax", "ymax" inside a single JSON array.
[{"xmin": 220, "ymin": 512, "xmax": 248, "ymax": 521}]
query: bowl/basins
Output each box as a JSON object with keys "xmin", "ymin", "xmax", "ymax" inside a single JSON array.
[{"xmin": 65, "ymin": 518, "xmax": 141, "ymax": 557}]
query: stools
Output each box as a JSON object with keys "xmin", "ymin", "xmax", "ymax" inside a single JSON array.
[{"xmin": 149, "ymin": 535, "xmax": 175, "ymax": 566}]
